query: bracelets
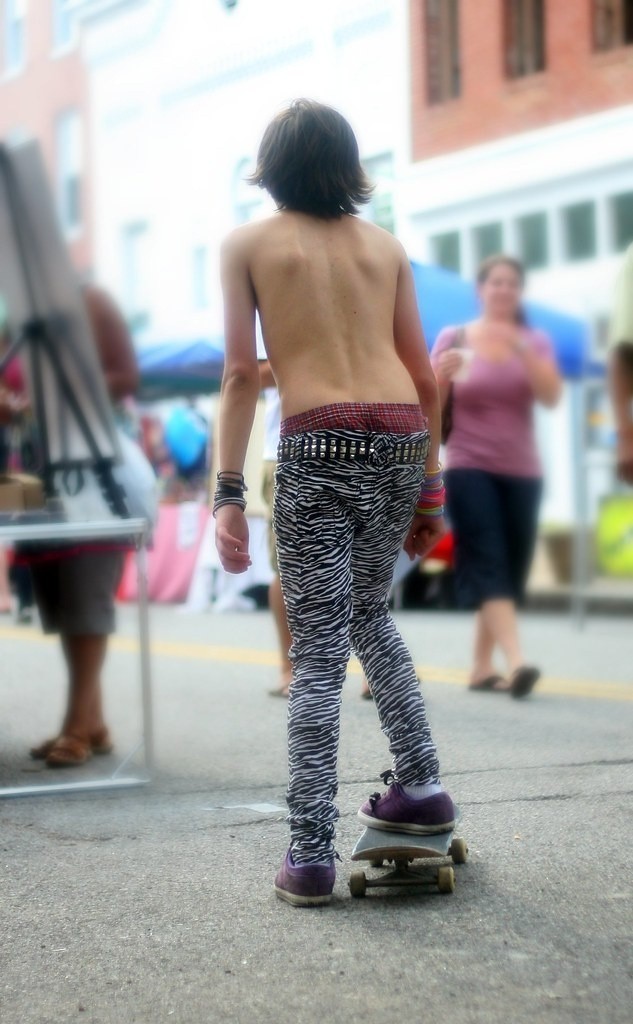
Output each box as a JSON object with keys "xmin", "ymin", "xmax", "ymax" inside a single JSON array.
[
  {"xmin": 213, "ymin": 470, "xmax": 248, "ymax": 519},
  {"xmin": 415, "ymin": 461, "xmax": 445, "ymax": 516},
  {"xmin": 514, "ymin": 337, "xmax": 528, "ymax": 355}
]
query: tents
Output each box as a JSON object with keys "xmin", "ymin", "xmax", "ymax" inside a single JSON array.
[{"xmin": 133, "ymin": 256, "xmax": 607, "ymax": 653}]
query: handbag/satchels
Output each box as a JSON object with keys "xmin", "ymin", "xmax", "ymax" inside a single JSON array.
[{"xmin": 441, "ymin": 379, "xmax": 456, "ymax": 447}]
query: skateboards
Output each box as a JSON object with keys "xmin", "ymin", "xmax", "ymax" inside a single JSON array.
[{"xmin": 346, "ymin": 805, "xmax": 470, "ymax": 898}]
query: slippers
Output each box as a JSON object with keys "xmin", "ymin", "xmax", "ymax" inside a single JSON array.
[
  {"xmin": 506, "ymin": 663, "xmax": 543, "ymax": 700},
  {"xmin": 469, "ymin": 671, "xmax": 510, "ymax": 692}
]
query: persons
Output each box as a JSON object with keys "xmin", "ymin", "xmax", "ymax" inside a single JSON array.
[
  {"xmin": 213, "ymin": 103, "xmax": 457, "ymax": 909},
  {"xmin": 430, "ymin": 256, "xmax": 562, "ymax": 700},
  {"xmin": 606, "ymin": 242, "xmax": 633, "ymax": 488},
  {"xmin": 254, "ymin": 307, "xmax": 423, "ymax": 701},
  {"xmin": 0, "ymin": 282, "xmax": 141, "ymax": 770}
]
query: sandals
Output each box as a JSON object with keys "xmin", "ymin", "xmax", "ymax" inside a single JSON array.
[
  {"xmin": 268, "ymin": 681, "xmax": 295, "ymax": 699},
  {"xmin": 48, "ymin": 732, "xmax": 94, "ymax": 765},
  {"xmin": 31, "ymin": 724, "xmax": 114, "ymax": 760},
  {"xmin": 359, "ymin": 677, "xmax": 424, "ymax": 702}
]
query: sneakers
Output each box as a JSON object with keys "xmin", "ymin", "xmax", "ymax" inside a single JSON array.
[
  {"xmin": 273, "ymin": 841, "xmax": 339, "ymax": 908},
  {"xmin": 355, "ymin": 782, "xmax": 457, "ymax": 837}
]
